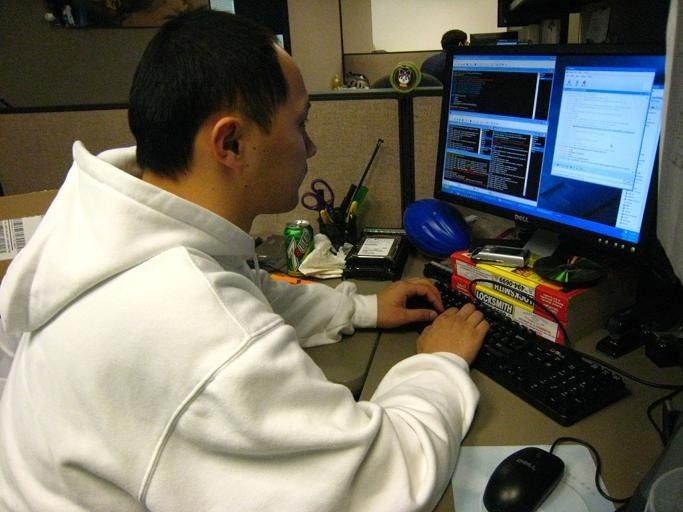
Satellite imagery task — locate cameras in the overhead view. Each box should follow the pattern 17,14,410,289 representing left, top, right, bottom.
477,244,530,268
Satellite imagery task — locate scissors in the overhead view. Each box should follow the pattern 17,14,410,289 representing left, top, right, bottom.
300,179,334,213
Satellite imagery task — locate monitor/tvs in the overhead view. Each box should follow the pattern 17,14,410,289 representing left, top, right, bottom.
470,32,518,46
433,41,666,259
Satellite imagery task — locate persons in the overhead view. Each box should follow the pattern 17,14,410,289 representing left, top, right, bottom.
0,5,491,512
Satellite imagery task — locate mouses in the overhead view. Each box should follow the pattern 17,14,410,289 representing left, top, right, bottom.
483,448,564,512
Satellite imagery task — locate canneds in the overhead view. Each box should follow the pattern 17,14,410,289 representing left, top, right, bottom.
284,219,316,278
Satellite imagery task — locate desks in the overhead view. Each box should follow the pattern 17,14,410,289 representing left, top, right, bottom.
358,252,683,512
256,233,396,396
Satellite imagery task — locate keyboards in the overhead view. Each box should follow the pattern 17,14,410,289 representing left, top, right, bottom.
405,282,626,426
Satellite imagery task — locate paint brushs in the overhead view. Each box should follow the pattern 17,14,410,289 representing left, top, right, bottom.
352,138,384,200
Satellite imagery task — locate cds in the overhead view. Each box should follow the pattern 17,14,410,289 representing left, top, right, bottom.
533,255,604,284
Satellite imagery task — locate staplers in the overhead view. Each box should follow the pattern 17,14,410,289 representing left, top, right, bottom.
595,296,681,359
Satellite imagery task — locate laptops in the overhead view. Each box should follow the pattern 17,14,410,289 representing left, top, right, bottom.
496,40,518,46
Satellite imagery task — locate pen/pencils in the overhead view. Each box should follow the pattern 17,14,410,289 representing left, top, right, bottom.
320,183,369,224
